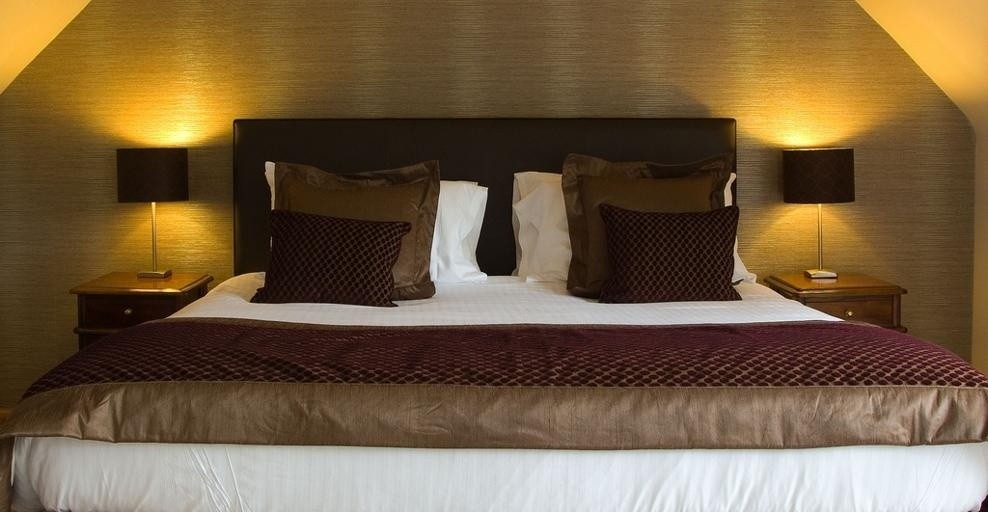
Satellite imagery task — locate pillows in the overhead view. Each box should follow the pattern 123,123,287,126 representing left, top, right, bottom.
269,158,442,299
505,165,758,287
260,154,488,283
594,197,745,308
247,208,415,310
559,148,736,300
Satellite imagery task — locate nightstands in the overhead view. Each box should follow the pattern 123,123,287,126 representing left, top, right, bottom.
764,273,911,336
68,270,213,353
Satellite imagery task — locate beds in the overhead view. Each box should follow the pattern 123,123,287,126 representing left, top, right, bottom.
0,117,987,512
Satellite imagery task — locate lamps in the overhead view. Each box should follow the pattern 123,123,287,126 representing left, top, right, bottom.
111,145,192,277
775,143,858,285
